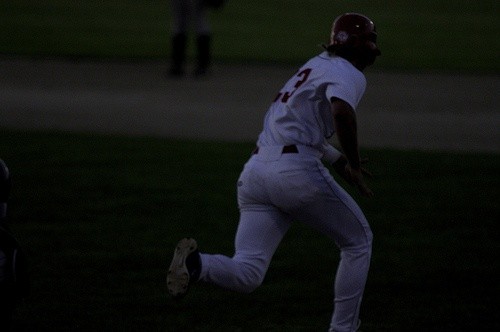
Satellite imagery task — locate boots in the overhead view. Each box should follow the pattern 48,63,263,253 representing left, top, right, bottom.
171,33,185,77
193,35,211,77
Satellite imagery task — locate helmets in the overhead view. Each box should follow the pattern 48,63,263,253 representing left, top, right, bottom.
330,12,377,64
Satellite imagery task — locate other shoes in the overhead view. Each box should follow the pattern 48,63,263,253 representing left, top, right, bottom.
166,237,198,296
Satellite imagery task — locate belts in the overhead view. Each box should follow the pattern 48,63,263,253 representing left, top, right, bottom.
251,144,299,153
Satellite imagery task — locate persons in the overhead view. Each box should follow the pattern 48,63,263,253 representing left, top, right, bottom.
166,14,379,332
169,0,217,78
